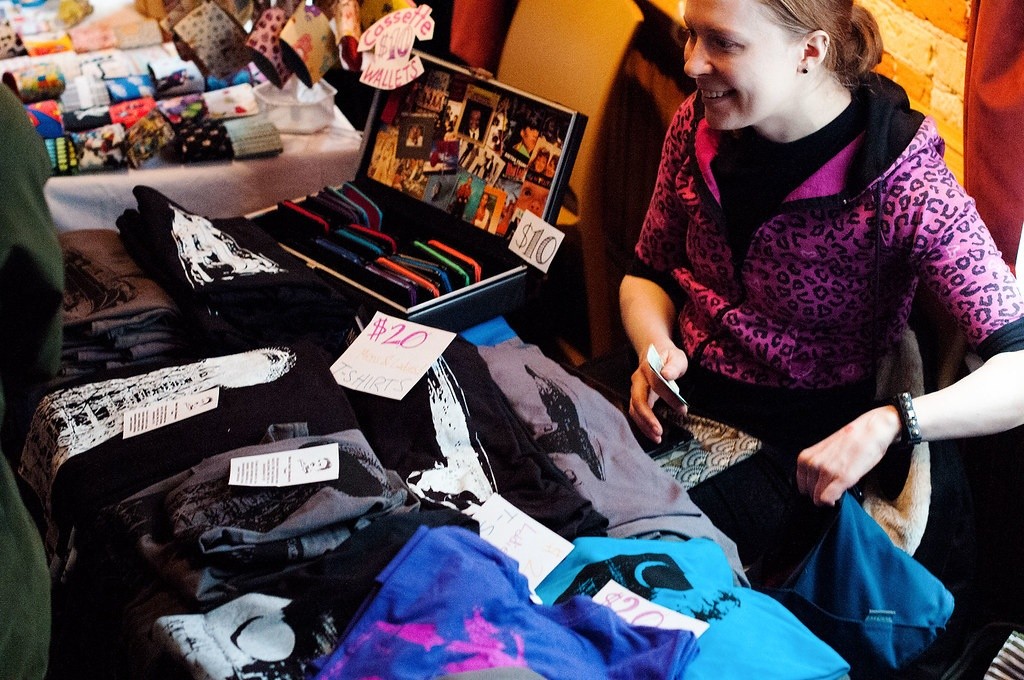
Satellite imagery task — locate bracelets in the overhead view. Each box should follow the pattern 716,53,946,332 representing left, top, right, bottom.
892,393,922,447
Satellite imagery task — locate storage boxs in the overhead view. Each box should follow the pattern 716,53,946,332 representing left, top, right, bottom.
230,44,600,338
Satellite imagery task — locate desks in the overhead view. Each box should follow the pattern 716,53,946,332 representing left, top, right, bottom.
41,94,366,237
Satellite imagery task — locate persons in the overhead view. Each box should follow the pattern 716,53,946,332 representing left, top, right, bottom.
297,455,332,475
467,109,485,143
618,0,1024,557
399,158,425,201
458,139,504,185
512,120,541,157
529,146,560,188
450,176,474,217
406,123,424,148
514,180,550,219
486,116,504,152
0,82,65,680
468,193,491,230
437,105,459,132
542,116,561,144
419,81,450,111
